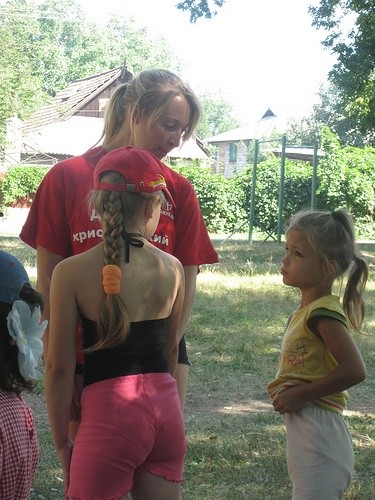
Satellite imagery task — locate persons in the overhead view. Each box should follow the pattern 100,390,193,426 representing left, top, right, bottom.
18,68,219,422
43,144,189,500
267,203,370,500
0,248,49,499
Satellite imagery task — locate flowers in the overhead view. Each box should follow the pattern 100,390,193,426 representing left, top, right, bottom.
6,299,49,381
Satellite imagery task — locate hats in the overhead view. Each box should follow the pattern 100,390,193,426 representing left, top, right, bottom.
0,250,31,305
92,144,176,212
